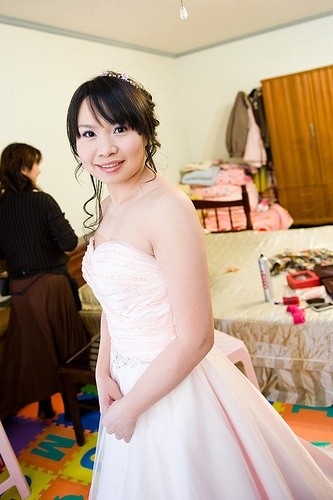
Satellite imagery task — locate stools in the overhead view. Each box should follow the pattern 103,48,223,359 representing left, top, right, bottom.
60,331,103,446
214,328,258,390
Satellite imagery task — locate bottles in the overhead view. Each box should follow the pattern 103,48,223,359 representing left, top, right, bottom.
259,254,272,302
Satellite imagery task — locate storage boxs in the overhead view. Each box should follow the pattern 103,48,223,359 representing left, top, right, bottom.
287,270,320,290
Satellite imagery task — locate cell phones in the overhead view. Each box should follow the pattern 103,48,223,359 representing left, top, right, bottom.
311,302,333,313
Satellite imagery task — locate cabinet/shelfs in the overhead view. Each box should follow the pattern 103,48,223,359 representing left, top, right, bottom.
259,65,333,225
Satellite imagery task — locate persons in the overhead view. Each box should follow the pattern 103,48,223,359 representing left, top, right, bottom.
0,142,89,419
67,73,333,500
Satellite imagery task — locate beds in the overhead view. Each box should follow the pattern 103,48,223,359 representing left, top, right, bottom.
189,164,296,231
0,224,333,407
